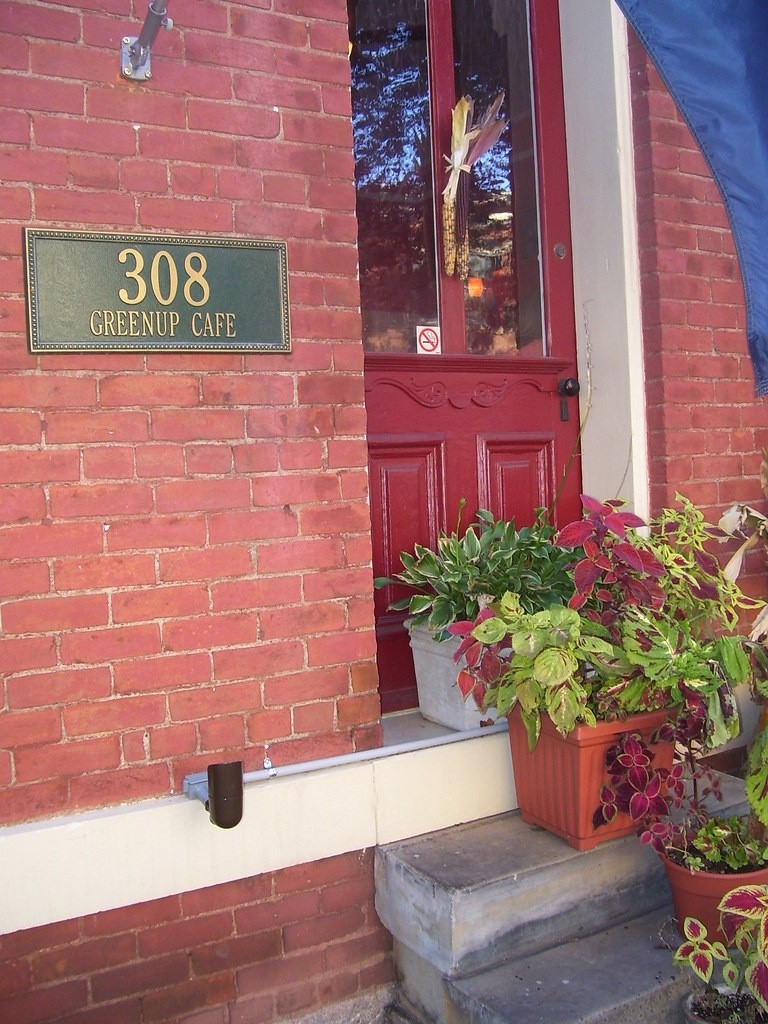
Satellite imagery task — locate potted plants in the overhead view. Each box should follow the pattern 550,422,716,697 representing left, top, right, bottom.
375,497,768,1024
